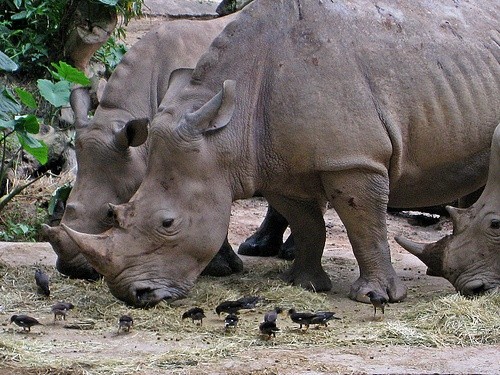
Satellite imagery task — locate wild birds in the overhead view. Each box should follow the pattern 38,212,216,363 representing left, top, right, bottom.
215,296,258,318
117,315,133,335
362,290,390,316
49,302,75,325
182,307,206,327
286,308,341,330
10,315,43,331
225,315,239,332
34,268,50,297
259,307,283,338
